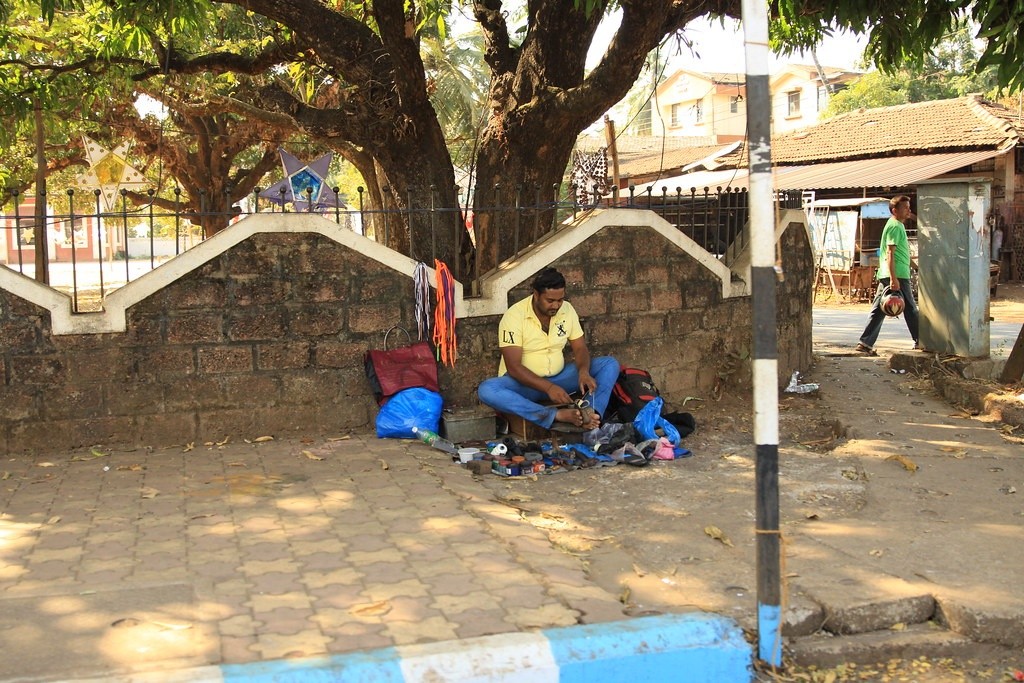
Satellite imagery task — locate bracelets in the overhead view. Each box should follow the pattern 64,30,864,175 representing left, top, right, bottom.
546,383,554,393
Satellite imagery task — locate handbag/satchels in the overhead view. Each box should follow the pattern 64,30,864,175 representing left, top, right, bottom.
376,387,443,439
363,324,440,406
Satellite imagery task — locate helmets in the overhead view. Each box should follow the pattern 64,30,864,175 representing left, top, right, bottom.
880,285,905,316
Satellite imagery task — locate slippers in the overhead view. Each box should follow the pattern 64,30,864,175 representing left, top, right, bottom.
635,438,661,460
607,441,648,467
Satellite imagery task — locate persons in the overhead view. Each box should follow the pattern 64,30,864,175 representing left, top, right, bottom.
857,194,924,354
478,268,620,429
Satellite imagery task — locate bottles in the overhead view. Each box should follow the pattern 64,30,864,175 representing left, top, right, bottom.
797,383,818,393
412,427,454,454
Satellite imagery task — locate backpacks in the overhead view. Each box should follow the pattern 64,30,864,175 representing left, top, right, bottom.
604,366,666,423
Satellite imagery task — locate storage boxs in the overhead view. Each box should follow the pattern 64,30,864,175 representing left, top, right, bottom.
440,406,497,444
502,394,585,442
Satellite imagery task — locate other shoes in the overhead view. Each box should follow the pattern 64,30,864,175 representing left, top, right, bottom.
915,342,919,348
855,342,877,356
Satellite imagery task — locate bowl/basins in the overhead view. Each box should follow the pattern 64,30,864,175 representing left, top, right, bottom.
458,448,480,463
